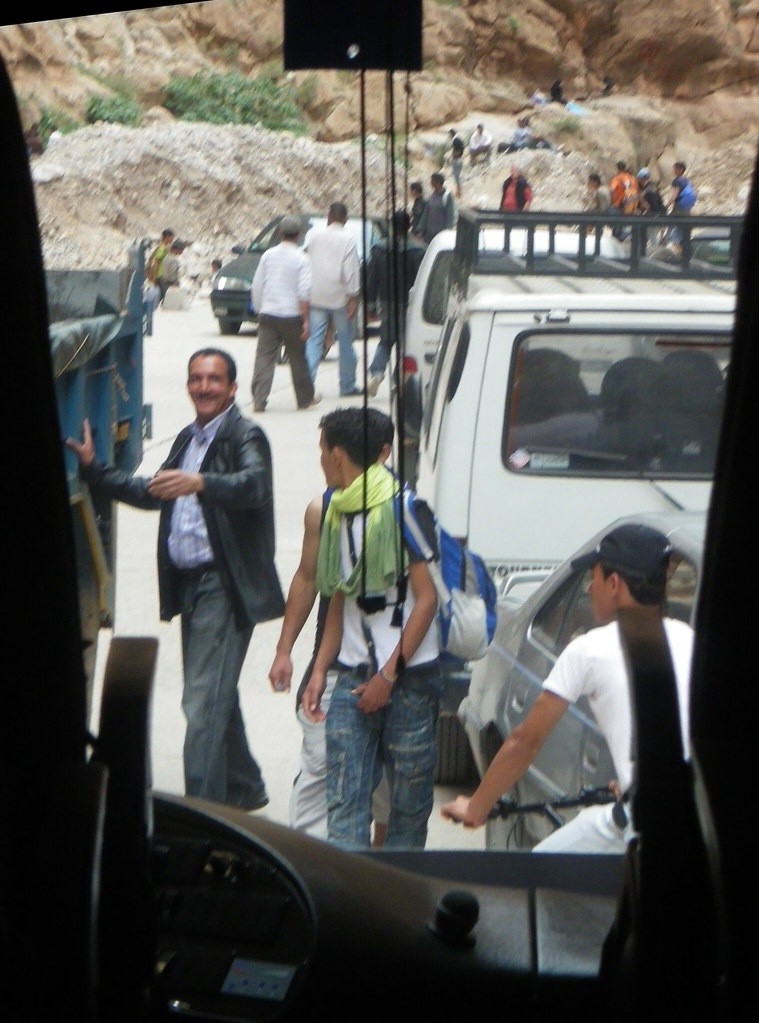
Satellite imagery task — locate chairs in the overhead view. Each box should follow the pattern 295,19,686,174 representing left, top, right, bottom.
517,348,724,425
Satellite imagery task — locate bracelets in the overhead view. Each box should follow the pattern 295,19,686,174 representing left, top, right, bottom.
381,668,397,685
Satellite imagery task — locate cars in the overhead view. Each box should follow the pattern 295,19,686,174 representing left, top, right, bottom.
208,211,400,335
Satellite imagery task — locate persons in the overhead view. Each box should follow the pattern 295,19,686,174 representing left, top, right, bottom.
303,409,445,851
146,229,221,309
364,211,428,398
269,409,393,846
580,160,697,248
302,202,367,396
250,216,323,412
501,168,534,212
441,522,695,853
447,128,466,198
497,78,569,154
67,348,287,813
466,124,495,166
410,174,456,243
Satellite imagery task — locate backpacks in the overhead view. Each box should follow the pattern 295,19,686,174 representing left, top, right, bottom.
322,484,496,669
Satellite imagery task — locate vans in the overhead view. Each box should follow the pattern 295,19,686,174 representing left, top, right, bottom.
393,227,625,488
399,268,738,782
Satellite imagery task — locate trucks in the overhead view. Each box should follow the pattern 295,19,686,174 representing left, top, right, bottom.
46,234,152,716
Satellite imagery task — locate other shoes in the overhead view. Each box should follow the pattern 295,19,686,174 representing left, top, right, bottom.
306,393,321,408
253,402,266,412
346,387,363,395
233,786,270,812
366,373,384,396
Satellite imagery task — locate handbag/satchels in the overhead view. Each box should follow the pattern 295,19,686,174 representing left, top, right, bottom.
675,178,696,212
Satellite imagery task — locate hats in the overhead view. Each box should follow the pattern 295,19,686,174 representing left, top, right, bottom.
636,168,649,179
571,524,671,573
281,214,304,236
